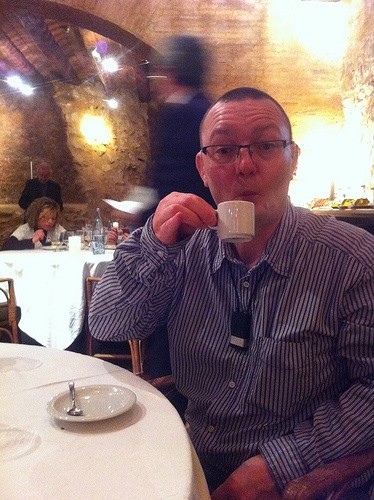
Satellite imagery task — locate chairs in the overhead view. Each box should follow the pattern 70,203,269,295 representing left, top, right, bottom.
145,375,374,500
86,275,145,376
0,277,20,344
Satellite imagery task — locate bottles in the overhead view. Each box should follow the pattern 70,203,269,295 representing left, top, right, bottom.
91,208,105,235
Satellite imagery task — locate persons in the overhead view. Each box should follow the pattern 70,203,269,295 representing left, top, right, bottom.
18,161,64,224
87,87,374,500
0,197,73,251
139,34,215,225
102,186,160,246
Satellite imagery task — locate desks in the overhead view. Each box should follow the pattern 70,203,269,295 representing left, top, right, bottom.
0,248,116,350
312,208,374,234
1,341,211,500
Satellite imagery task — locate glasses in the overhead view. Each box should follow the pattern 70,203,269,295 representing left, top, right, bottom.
199,139,292,165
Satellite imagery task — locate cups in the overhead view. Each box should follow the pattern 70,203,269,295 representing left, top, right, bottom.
208,201,255,243
62,230,92,246
68,236,82,254
92,235,105,254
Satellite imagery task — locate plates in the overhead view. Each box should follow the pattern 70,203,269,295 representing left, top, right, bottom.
105,244,116,250
47,383,136,422
40,246,67,250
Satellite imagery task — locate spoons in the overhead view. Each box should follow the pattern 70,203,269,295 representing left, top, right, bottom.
66,380,83,416
